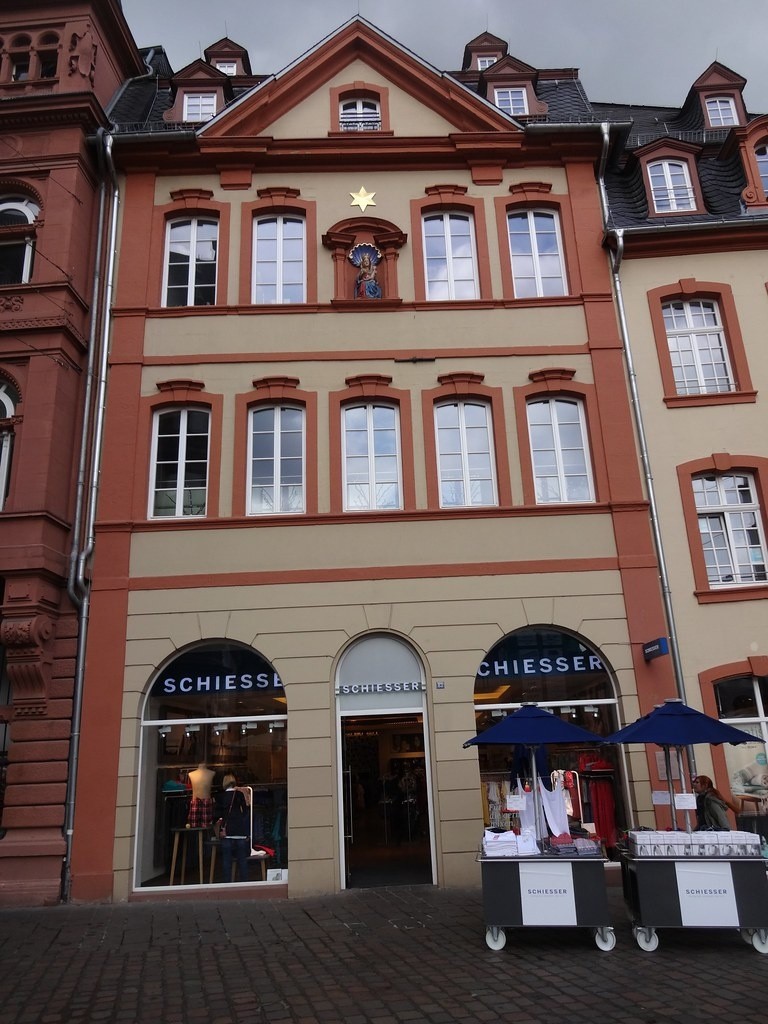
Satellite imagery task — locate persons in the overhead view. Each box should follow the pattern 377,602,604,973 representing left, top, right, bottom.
693,775,740,830
360,252,376,273
213,774,250,882
188,763,216,828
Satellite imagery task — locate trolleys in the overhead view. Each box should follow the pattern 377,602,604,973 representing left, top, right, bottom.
615,841,767,954
475,847,616,953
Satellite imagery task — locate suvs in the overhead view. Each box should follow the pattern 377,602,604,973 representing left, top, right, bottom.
154,761,287,830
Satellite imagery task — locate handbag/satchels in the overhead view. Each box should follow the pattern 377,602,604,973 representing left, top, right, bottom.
212,818,227,838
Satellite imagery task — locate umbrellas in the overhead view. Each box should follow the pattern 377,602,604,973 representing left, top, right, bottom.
464,702,604,842
606,698,766,832
